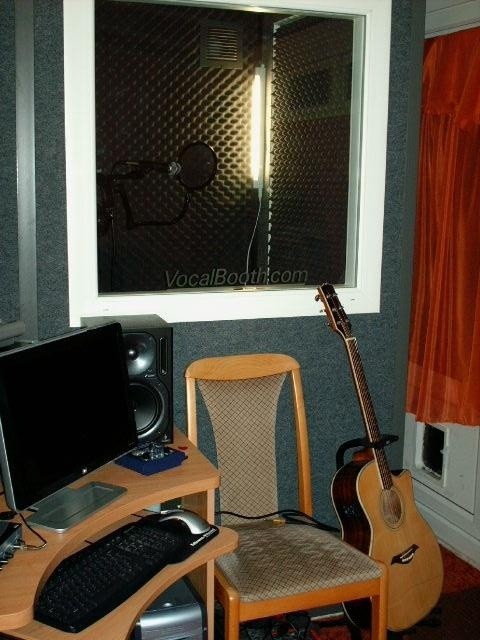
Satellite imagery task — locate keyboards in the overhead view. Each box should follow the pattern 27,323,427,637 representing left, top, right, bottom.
36,522,187,634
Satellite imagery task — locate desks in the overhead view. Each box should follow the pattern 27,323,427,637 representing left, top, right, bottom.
0,424,239,640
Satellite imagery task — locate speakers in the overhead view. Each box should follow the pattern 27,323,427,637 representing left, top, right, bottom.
79,314,174,452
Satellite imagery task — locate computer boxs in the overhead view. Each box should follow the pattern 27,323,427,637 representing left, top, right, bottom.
131,578,204,640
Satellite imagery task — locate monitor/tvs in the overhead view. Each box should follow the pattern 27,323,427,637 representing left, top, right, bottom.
0,320,140,535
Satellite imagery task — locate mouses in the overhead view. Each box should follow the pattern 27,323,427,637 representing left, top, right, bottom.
156,509,212,537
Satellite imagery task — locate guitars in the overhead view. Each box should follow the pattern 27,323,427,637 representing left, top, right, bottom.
315,283,444,636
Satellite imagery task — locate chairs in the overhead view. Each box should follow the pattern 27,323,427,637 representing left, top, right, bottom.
185,353,389,639
